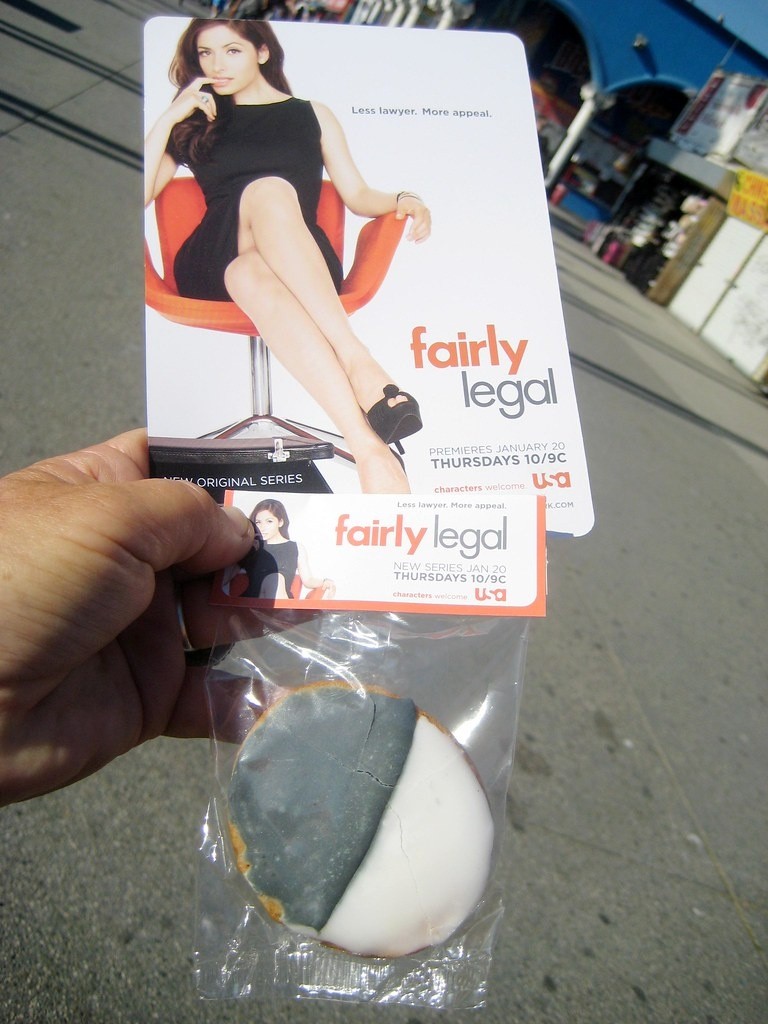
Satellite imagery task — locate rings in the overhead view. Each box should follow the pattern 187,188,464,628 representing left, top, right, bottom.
202,96,209,102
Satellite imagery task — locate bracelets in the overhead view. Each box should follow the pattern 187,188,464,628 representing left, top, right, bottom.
397,191,420,201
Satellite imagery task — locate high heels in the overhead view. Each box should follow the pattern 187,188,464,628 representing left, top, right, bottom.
365,384,422,454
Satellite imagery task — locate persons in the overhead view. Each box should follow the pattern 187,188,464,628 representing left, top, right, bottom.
0,427,336,807
143,18,432,494
222,499,329,599
